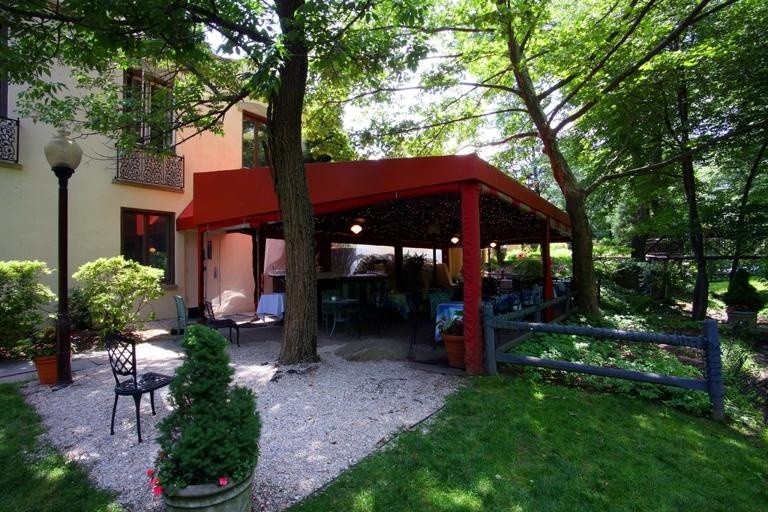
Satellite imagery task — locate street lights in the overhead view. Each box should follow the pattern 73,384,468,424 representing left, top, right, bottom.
43,126,83,384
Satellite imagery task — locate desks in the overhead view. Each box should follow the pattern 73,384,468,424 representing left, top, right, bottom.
256,293,286,326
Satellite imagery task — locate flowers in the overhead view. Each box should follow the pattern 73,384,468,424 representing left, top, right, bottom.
146,322,263,498
433,310,464,351
30,326,58,361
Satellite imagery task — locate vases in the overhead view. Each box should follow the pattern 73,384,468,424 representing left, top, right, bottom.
161,470,254,512
441,332,465,369
34,355,58,384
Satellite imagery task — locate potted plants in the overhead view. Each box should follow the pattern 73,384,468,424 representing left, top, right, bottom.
709,268,767,332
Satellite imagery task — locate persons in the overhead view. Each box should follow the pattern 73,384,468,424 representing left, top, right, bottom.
448,269,464,302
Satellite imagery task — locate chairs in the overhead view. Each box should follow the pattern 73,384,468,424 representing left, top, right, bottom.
105,329,176,443
205,300,240,347
172,295,204,346
